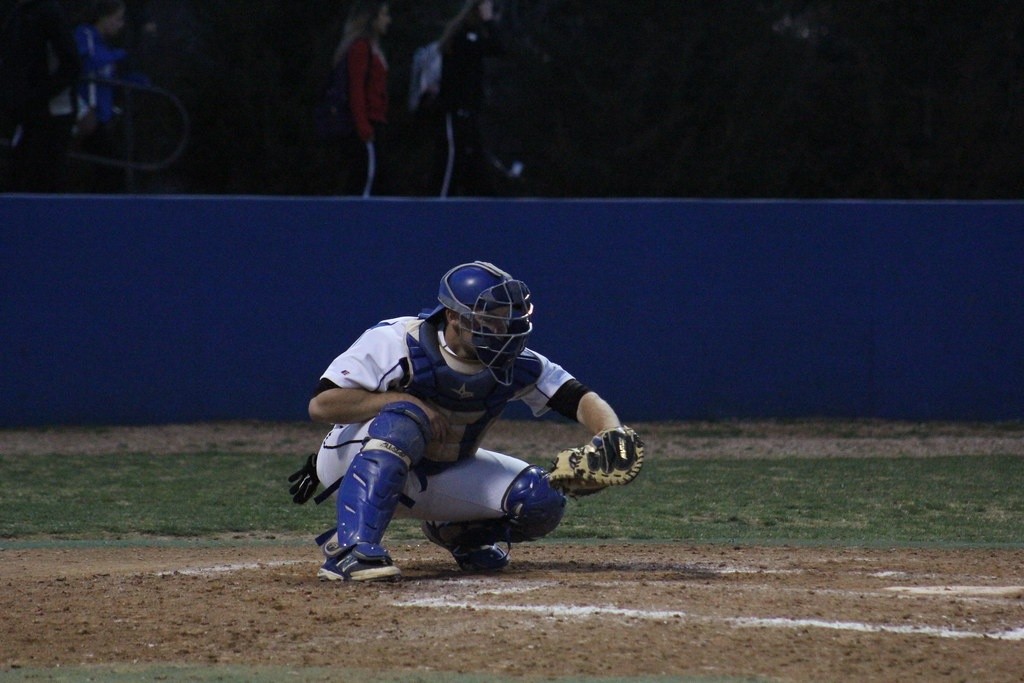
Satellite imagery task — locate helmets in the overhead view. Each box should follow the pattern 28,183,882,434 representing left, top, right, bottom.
425,261,516,321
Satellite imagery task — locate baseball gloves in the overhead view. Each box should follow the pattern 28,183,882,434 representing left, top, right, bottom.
547,424,645,497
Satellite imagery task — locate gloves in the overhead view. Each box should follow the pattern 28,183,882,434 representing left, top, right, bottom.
288,453,320,505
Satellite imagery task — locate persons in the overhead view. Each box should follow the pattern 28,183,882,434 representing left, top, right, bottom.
0,0,152,195
337,0,393,200
308,259,644,583
407,0,527,198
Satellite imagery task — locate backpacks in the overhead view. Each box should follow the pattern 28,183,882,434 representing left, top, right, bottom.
312,53,352,141
408,37,444,121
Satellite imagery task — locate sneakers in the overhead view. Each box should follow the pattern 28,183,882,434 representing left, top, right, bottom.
421,519,503,575
317,542,401,584
